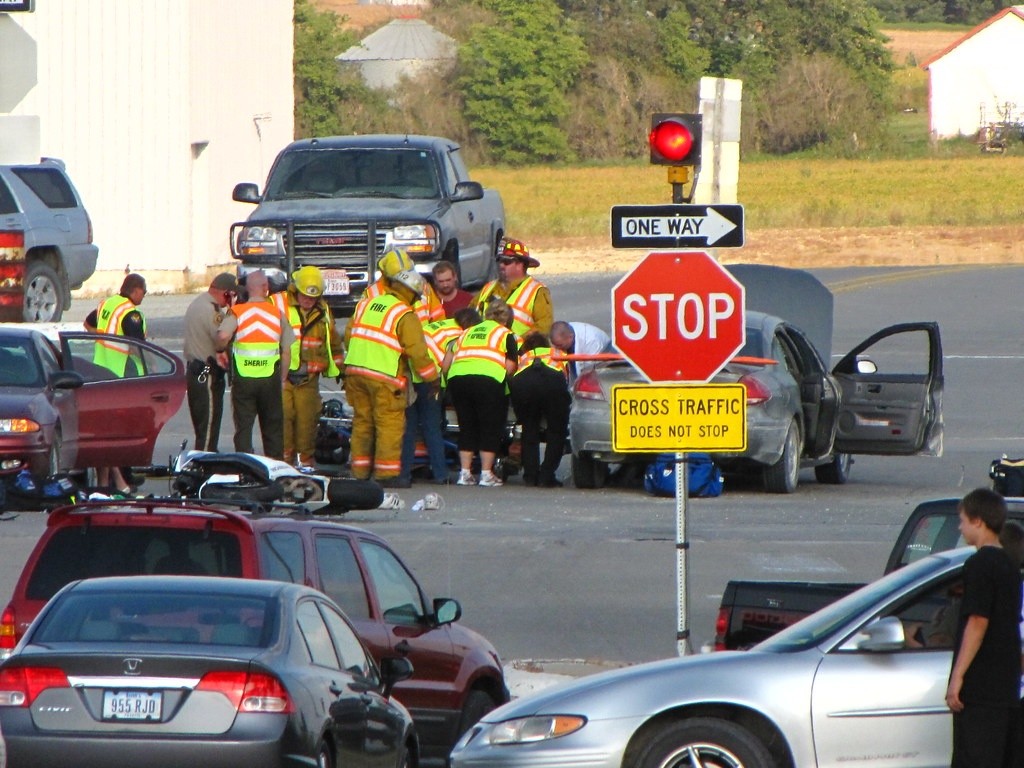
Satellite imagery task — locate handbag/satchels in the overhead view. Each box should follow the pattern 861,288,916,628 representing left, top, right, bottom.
643,450,725,498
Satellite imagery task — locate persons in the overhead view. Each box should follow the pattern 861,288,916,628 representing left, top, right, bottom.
181,273,235,452
268,264,344,473
944,487,1024,768
344,269,439,488
18,343,132,497
344,248,446,463
84,274,150,491
399,237,611,488
212,269,293,462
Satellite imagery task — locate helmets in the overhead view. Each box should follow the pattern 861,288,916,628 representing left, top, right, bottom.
292,265,325,297
378,247,415,282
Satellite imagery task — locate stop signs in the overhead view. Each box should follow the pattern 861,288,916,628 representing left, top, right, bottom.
611,249,746,384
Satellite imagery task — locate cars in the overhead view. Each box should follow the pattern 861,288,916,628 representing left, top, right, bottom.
0,573,421,768
569,308,945,493
0,495,512,763
447,544,1024,768
1,329,187,508
1,156,99,325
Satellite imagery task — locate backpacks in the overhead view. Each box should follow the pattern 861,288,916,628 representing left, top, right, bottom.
0,469,136,517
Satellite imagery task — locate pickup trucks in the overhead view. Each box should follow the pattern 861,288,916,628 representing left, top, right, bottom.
231,133,507,315
711,492,1024,651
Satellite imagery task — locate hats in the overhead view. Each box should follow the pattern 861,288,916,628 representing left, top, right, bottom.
210,272,242,297
392,269,424,300
494,238,540,269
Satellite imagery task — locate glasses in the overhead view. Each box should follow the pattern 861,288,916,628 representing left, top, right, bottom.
141,287,147,295
497,257,521,265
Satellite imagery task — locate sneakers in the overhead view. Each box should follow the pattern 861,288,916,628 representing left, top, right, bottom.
479,472,504,486
456,471,477,485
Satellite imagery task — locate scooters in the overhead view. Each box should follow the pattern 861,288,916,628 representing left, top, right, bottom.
165,438,385,519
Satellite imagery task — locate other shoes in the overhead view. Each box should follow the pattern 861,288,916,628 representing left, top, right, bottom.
436,470,458,485
525,480,535,487
374,475,411,488
123,486,146,498
536,480,563,488
